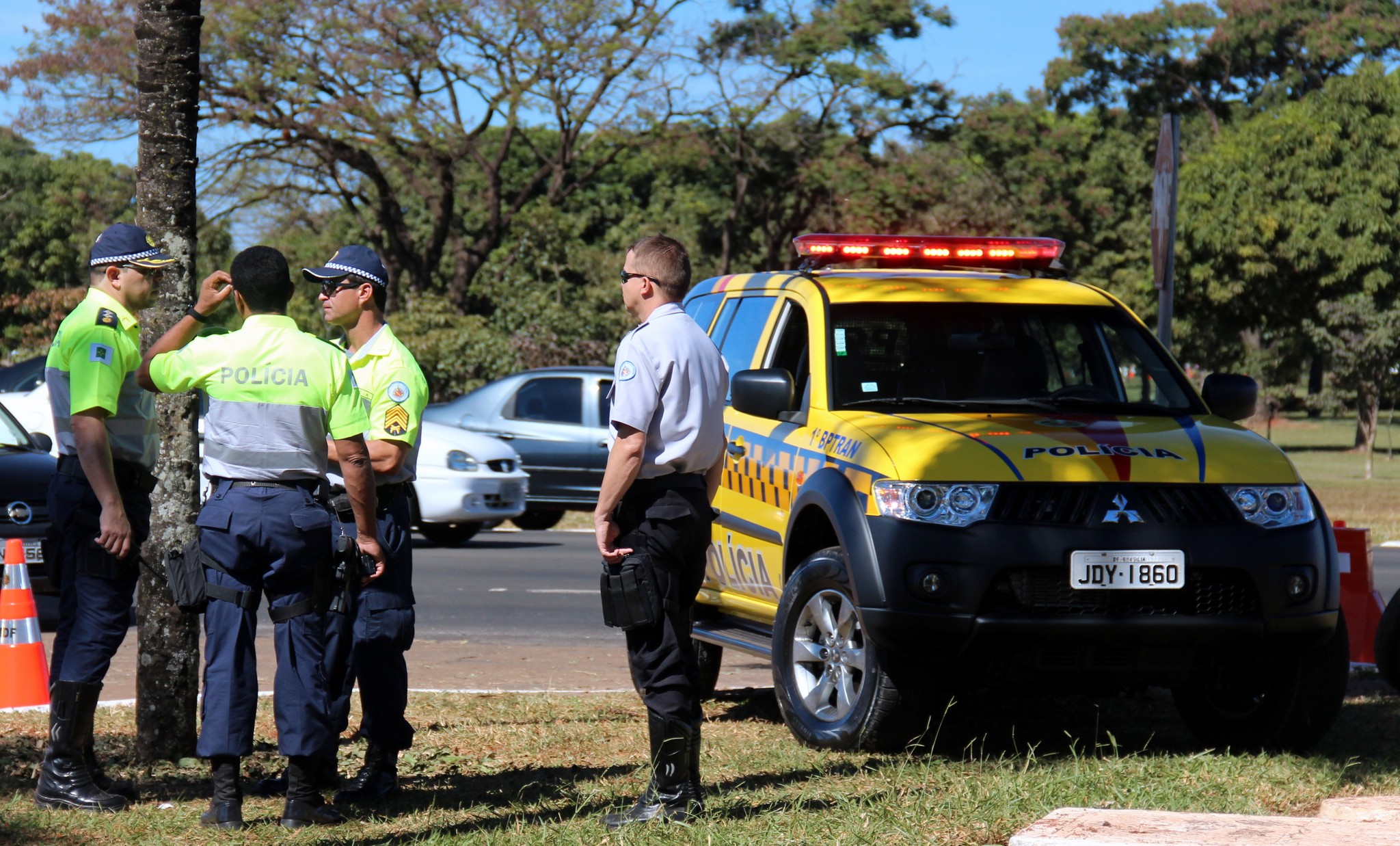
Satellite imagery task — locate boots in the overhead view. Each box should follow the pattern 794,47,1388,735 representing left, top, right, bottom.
280,754,349,828
199,755,244,831
597,708,704,828
33,676,132,813
333,748,400,806
259,752,338,793
688,723,704,817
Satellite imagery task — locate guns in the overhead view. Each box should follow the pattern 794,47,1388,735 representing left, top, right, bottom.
327,534,378,613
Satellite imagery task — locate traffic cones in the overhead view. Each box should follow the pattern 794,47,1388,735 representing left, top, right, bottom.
0,535,50,714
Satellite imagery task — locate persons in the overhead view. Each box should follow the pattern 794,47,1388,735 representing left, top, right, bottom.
133,245,385,831
35,222,181,813
594,237,728,828
301,246,430,817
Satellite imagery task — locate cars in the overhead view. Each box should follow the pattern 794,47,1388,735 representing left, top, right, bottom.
198,397,530,549
0,352,65,634
678,230,1352,757
420,364,614,530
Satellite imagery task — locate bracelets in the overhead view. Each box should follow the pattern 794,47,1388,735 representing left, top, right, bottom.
183,304,207,324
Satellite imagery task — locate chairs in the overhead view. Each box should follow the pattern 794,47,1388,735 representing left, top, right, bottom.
956,330,1050,398
798,327,871,411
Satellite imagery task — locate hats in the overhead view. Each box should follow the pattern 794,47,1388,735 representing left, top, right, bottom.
301,244,389,289
88,221,180,268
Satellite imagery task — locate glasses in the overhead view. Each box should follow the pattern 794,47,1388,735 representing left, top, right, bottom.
621,269,662,286
322,279,365,297
